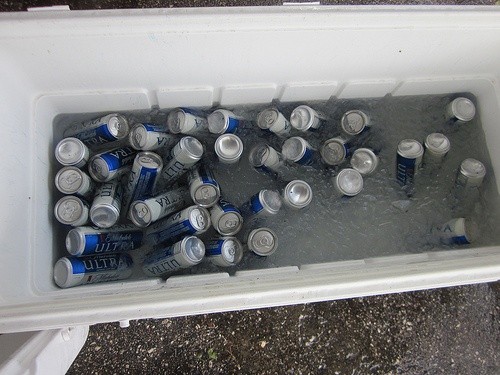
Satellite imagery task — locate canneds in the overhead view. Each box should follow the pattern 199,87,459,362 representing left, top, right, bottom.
395,138,425,199
423,132,450,172
432,218,475,247
53,106,381,290
440,97,476,127
454,158,486,204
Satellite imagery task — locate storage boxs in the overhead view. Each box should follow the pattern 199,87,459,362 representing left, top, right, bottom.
0,2,500,334
